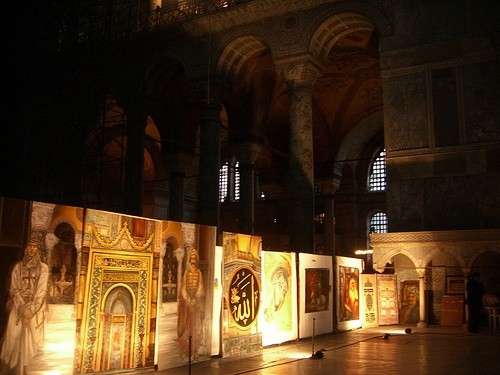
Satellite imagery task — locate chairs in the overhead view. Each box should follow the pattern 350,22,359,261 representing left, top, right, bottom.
485,307,500,331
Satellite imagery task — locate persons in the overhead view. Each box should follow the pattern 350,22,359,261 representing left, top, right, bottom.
467,272,486,334
